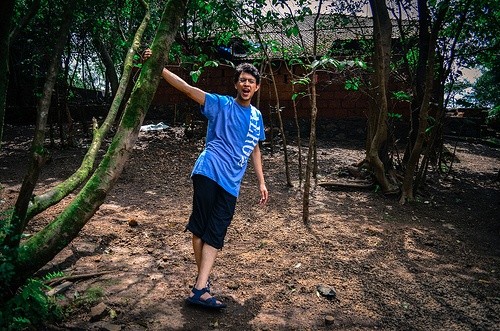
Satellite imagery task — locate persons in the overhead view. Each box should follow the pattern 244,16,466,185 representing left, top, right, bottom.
139,48,268,310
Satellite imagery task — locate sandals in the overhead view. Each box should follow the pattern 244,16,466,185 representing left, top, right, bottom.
186,286,226,308
192,276,211,293
